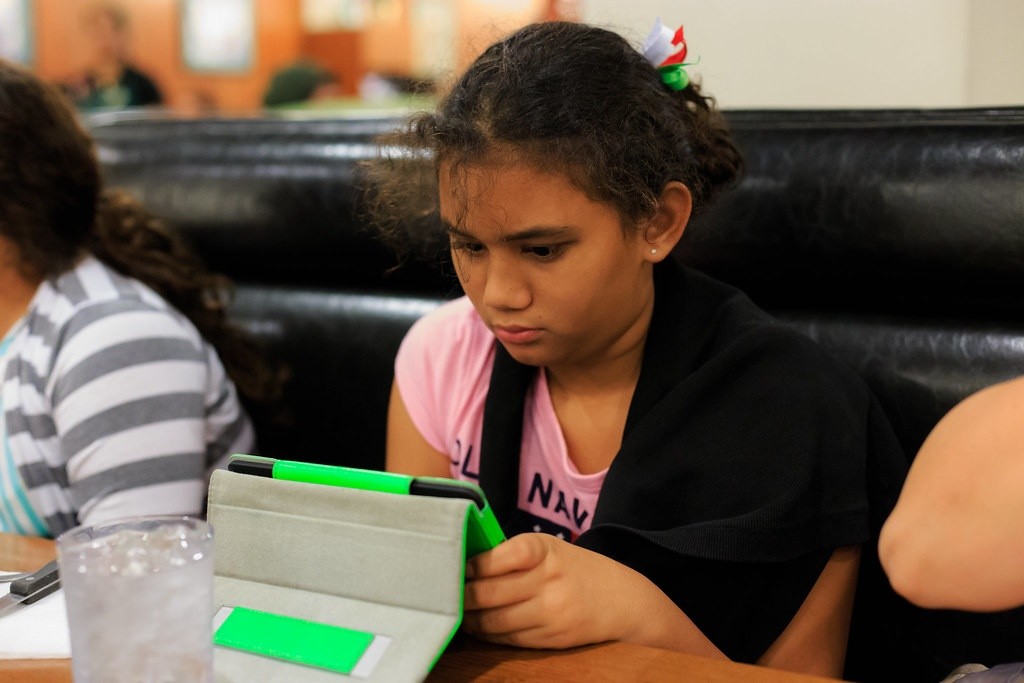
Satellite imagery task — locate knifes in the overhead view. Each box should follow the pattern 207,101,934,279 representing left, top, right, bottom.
1,558,59,616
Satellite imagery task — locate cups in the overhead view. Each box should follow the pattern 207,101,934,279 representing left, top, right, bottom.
58,515,216,683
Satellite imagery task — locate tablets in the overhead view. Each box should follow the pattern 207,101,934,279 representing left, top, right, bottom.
227,454,510,557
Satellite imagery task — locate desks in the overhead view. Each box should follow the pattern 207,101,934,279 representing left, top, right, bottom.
1,531,846,683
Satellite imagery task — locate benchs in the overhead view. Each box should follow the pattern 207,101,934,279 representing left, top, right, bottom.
84,103,1024,683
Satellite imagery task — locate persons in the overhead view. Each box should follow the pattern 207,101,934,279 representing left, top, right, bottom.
379,18,894,683
1,58,253,553
878,373,1021,612
